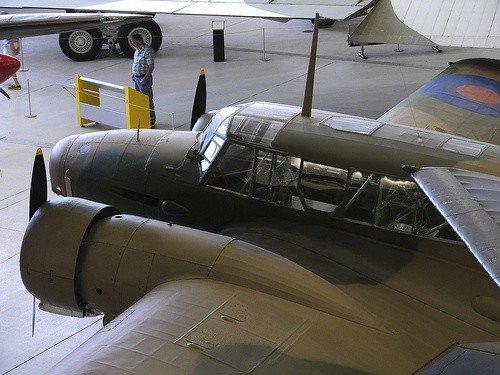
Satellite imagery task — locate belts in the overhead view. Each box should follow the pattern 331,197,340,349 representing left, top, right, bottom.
133,73,147,78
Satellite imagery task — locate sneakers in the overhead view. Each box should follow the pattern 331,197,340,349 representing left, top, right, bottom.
8,84,22,90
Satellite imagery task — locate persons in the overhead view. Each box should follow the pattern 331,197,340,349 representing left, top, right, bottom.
130,34,156,129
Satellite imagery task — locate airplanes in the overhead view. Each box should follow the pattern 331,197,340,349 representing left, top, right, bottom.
23,52,499,374
